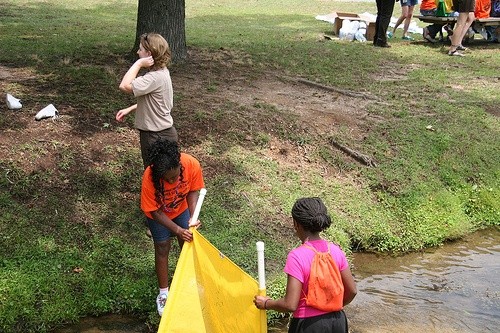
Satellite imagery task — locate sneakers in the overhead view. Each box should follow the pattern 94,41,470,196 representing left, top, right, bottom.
35,104,58,120
156,294,167,316
426,35,436,42
423,28,429,39
439,35,443,41
6,94,22,109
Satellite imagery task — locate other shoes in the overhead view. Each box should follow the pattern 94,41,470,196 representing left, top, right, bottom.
374,43,391,47
456,45,467,50
403,36,415,41
389,32,392,37
448,50,463,55
147,229,177,237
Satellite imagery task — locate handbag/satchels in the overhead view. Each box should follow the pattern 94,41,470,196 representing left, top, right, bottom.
435,1,445,16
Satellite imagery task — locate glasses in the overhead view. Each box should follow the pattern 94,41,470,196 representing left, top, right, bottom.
144,36,149,45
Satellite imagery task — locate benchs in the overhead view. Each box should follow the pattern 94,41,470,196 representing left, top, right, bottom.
413,15,500,43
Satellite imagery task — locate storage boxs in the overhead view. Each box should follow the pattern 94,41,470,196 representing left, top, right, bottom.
365,22,377,41
334,12,365,36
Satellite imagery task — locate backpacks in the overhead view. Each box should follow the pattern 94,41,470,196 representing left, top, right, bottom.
302,243,344,312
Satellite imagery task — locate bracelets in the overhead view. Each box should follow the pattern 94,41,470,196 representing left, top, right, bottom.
265,299,272,310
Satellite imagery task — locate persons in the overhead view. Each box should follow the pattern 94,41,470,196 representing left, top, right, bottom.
140,138,206,317
375,0,500,57
253,197,357,333
116,32,178,237
